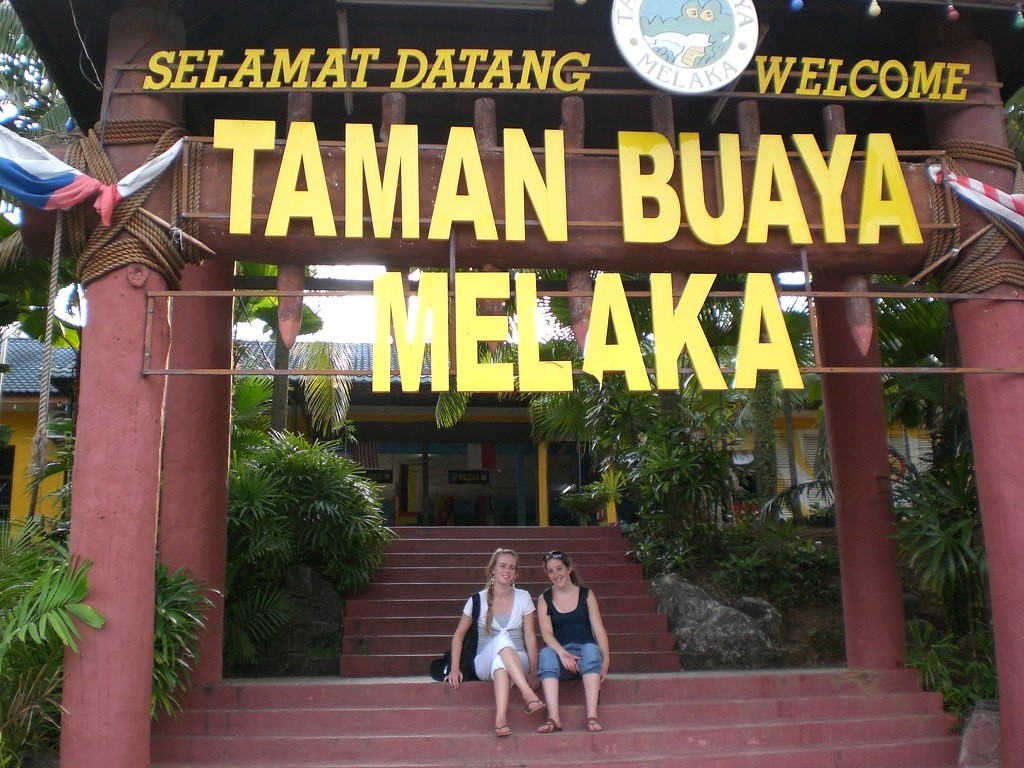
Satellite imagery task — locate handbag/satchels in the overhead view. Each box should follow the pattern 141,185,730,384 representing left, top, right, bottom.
430,592,481,682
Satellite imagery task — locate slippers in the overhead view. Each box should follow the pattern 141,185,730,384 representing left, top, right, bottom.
494,725,512,736
524,699,545,714
586,717,602,732
538,719,563,733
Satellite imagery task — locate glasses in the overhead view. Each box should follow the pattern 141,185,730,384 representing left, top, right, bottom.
542,552,564,568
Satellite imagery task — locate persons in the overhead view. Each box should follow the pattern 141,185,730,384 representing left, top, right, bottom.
536,550,610,734
443,547,546,737
